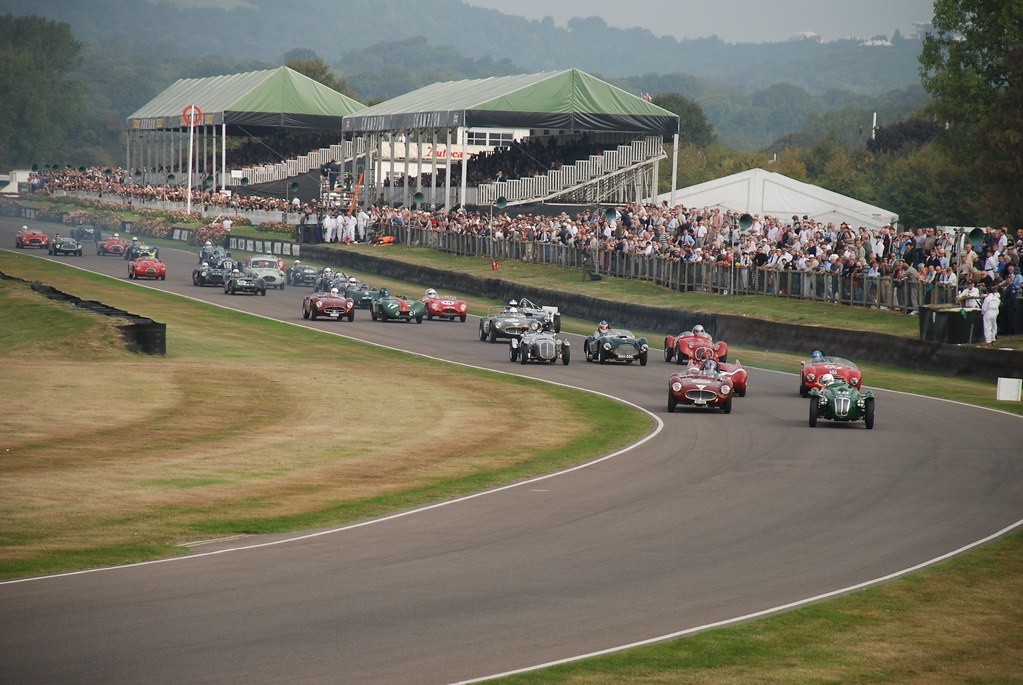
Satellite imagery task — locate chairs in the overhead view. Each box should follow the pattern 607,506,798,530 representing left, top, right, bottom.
688,367,701,374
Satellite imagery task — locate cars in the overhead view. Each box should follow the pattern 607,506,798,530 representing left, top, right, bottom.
668,358,735,413
72,223,103,242
808,373,878,431
479,302,543,343
128,251,168,280
49,235,83,257
584,326,650,366
287,259,424,326
127,242,160,261
242,254,288,290
191,240,242,288
507,320,574,366
301,289,356,323
97,236,128,257
17,229,50,250
421,292,468,323
517,298,563,333
689,354,748,395
220,263,267,296
663,325,729,363
799,348,864,395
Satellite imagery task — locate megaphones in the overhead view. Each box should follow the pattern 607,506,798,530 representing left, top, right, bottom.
734,214,753,229
494,196,507,208
288,181,299,192
31,163,175,180
412,192,424,200
599,207,617,220
204,176,213,184
962,227,984,246
238,177,248,185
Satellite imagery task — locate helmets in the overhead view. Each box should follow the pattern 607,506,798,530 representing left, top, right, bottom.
599,320,609,333
426,289,436,298
507,299,518,308
379,288,388,297
331,287,338,295
812,350,822,360
705,361,715,372
349,277,357,285
692,325,705,335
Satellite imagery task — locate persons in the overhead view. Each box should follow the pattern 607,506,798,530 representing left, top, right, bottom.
510,308,518,318
383,136,617,189
398,133,405,142
509,299,517,306
22,225,28,232
152,132,353,173
812,350,821,361
822,374,834,387
982,286,999,347
594,321,608,339
693,325,704,334
428,289,436,299
705,361,715,372
29,167,1023,317
700,352,710,361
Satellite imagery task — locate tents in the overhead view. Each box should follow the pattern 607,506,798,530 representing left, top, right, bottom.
125,65,369,191
341,68,680,211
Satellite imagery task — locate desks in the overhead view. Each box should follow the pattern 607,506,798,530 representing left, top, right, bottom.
918,304,985,345
